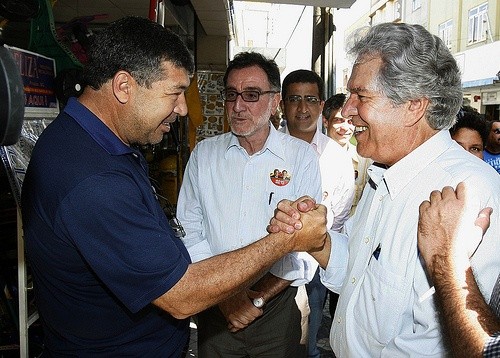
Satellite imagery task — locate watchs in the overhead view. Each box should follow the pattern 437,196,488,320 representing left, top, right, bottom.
249,297,267,310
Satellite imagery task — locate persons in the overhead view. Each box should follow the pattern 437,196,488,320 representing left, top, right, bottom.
22,16,327,358
183,23,500,358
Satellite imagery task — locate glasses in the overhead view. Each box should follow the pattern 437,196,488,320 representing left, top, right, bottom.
220,87,279,102
156,194,186,238
284,94,320,105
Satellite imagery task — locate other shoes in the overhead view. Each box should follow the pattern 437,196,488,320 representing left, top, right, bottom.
316,337,332,351
